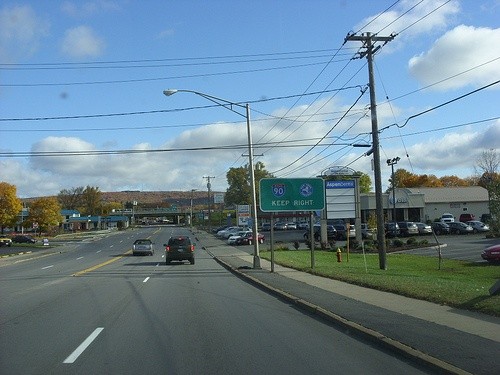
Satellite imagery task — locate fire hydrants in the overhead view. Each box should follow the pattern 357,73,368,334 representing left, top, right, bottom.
335,248,343,262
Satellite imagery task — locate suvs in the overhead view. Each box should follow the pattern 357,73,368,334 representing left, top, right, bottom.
460,214,474,224
440,213,456,223
479,214,493,223
164,236,195,265
385,222,400,238
361,224,378,238
430,222,450,235
334,225,357,237
334,226,346,240
304,225,337,240
397,222,419,236
447,222,473,234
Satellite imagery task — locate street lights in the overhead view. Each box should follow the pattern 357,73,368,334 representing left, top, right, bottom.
164,89,262,270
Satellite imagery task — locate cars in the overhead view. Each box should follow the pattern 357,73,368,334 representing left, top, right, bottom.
263,222,272,231
466,221,490,233
287,222,296,230
221,228,252,238
299,220,309,229
217,227,241,237
132,240,154,255
228,231,251,244
213,226,230,232
273,223,287,231
236,232,265,245
12,235,38,243
417,222,432,235
481,244,500,262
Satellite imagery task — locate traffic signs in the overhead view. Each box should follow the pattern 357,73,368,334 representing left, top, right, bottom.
258,177,326,212
